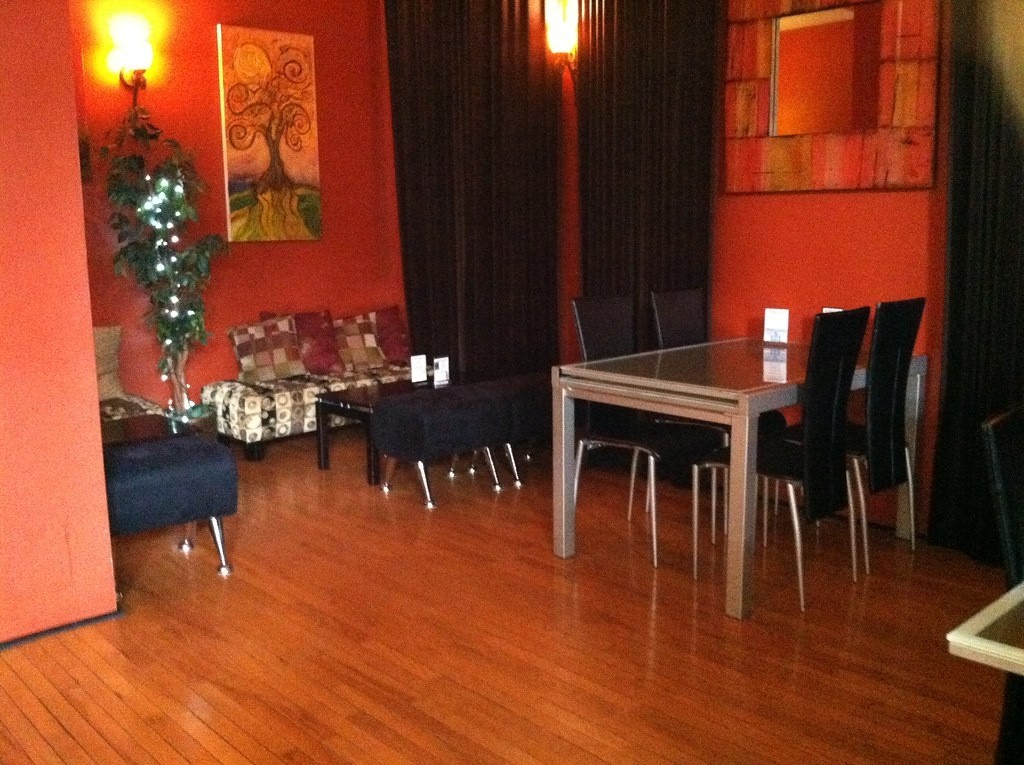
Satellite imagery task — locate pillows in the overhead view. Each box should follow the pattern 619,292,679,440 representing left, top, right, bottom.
332,312,390,371
260,309,346,376
376,305,411,368
227,317,305,378
93,325,123,405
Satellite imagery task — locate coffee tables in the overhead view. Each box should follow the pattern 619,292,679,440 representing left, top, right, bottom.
315,374,494,485
101,413,205,449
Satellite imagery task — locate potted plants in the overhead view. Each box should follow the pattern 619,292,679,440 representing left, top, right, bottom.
101,106,228,444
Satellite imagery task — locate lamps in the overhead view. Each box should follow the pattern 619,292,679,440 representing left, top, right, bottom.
99,16,152,106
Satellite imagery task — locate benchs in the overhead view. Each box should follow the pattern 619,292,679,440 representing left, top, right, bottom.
200,361,435,461
467,373,554,487
104,433,237,577
100,396,164,423
370,380,506,509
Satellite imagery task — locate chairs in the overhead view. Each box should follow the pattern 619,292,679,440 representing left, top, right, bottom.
572,294,717,567
693,305,870,610
776,296,926,580
644,289,787,512
980,403,1024,589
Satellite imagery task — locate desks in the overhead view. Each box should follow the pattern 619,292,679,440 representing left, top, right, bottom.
551,338,932,617
947,582,1024,765
